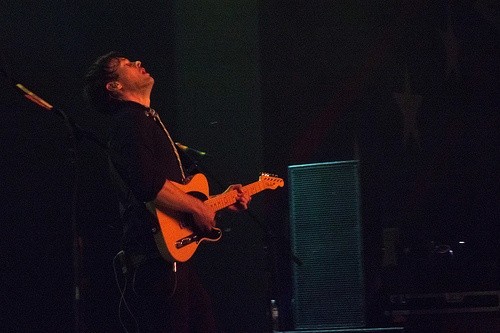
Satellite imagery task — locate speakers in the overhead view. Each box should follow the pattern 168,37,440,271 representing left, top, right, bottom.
286,159,368,330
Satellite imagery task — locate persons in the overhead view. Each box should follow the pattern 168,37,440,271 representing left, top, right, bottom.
85,49,252,333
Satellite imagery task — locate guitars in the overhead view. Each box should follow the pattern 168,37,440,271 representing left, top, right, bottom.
150,172,285,263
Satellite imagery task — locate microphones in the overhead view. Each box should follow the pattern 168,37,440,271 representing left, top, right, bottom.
174,143,208,158
16,85,66,118
